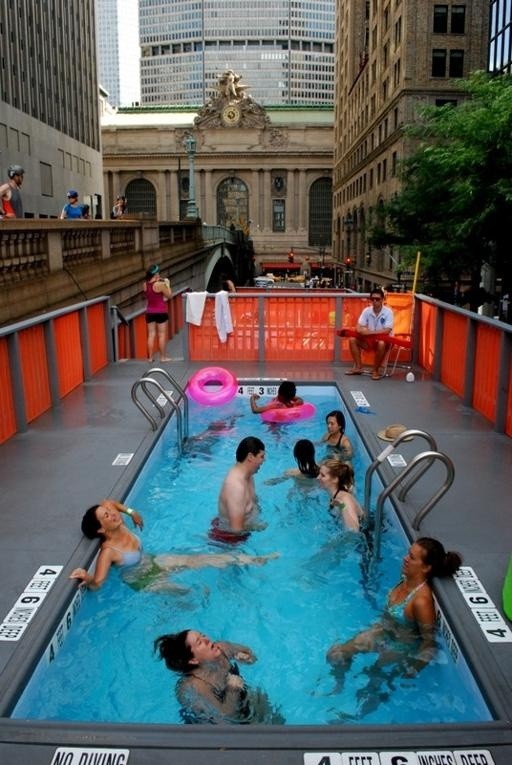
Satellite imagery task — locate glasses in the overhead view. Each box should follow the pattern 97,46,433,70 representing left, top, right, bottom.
370,297,381,302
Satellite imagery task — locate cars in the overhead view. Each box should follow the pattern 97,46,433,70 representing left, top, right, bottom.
255,272,332,289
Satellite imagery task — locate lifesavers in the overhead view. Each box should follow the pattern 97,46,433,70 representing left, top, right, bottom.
261,399,316,423
189,366,236,404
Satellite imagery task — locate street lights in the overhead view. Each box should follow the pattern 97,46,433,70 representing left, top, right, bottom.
235,194,241,227
184,134,201,220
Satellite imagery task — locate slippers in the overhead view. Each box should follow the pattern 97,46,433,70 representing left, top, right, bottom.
345,370,362,375
372,370,381,381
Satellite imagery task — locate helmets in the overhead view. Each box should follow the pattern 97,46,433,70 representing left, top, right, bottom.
8,165,24,179
67,190,79,198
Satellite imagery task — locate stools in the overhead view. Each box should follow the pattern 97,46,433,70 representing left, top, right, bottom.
356,328,394,380
389,330,417,377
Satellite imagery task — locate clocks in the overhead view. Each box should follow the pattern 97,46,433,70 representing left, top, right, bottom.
221,104,243,126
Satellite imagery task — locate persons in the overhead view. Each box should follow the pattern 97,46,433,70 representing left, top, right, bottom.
60,190,83,219
0,164,25,220
80,204,90,218
345,290,394,380
143,263,173,363
218,273,236,293
225,71,238,104
112,195,128,219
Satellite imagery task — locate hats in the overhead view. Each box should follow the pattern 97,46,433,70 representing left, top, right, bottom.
376,422,415,443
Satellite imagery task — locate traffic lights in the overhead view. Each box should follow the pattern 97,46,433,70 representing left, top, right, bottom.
291,252,294,263
347,258,350,265
288,252,290,262
